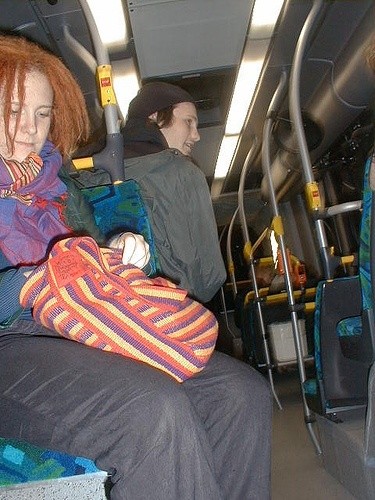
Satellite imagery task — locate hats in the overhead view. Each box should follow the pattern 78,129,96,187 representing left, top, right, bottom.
127,82,194,119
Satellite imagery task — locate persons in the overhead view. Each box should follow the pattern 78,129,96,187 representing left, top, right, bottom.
94,81,226,303
0,36,274,499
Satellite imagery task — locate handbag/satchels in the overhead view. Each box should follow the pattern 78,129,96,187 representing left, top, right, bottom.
20,235,219,383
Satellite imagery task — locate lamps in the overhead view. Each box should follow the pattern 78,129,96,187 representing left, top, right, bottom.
210,0,286,200
85,0,142,126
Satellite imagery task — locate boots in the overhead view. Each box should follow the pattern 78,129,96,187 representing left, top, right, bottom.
242,342,259,368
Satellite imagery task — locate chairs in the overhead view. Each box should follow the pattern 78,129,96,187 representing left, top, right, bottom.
302,274,372,416
0,435,108,499
334,154,375,366
78,179,157,280
